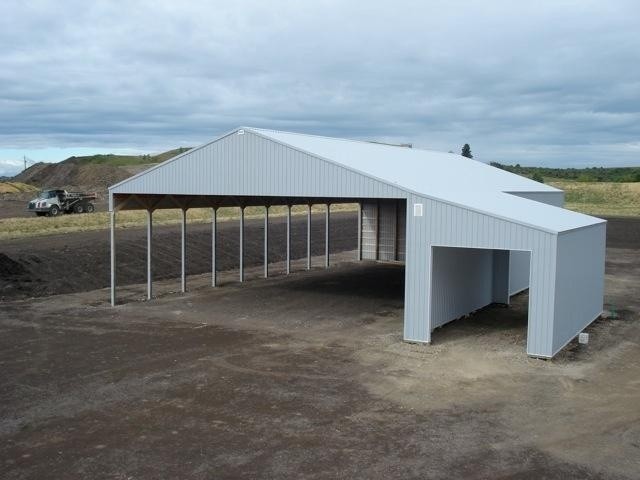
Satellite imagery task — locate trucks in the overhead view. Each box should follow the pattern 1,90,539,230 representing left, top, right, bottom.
25,189,97,217
